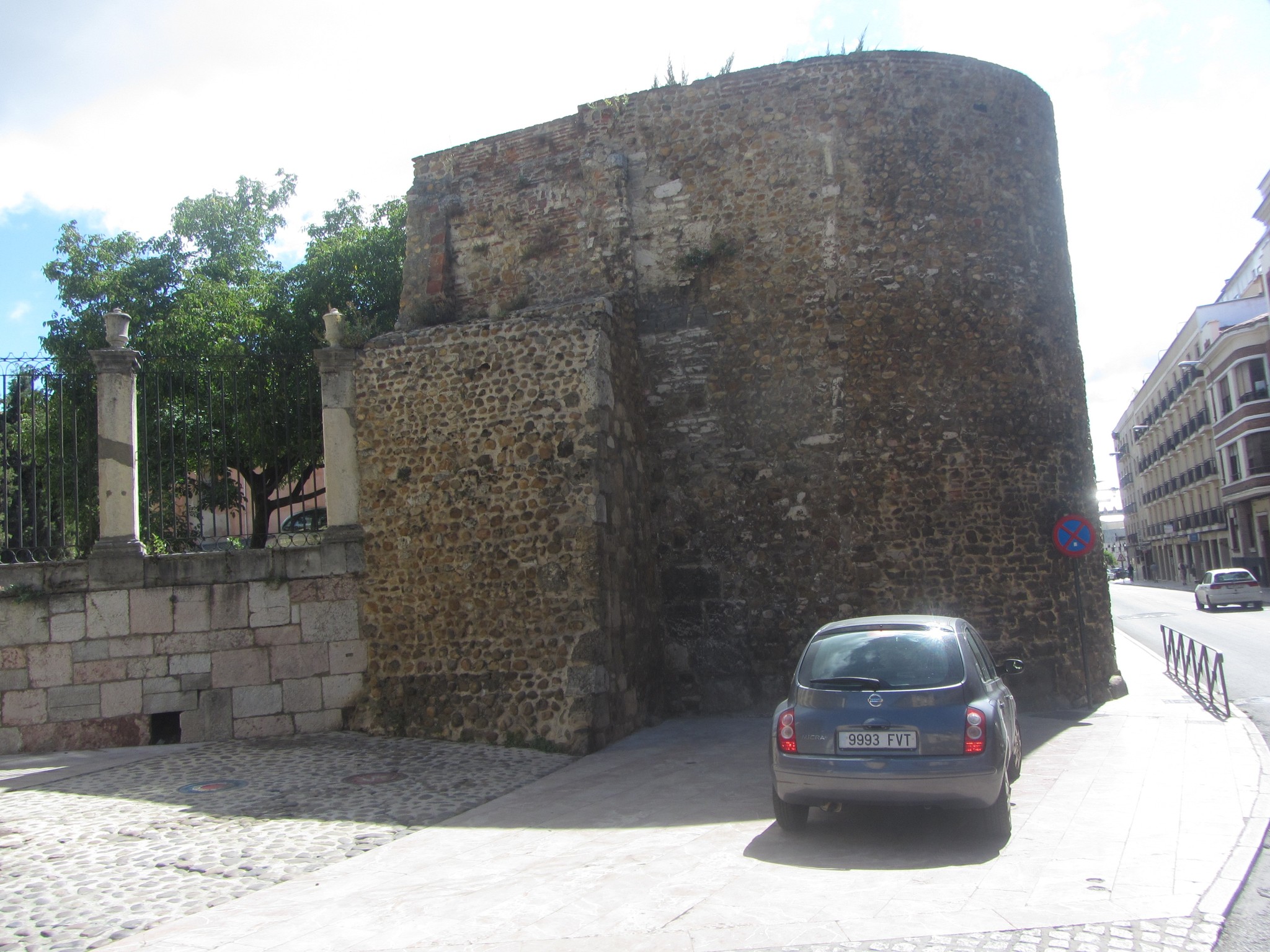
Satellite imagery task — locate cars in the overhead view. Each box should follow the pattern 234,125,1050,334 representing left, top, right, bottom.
1110,568,1129,579
768,615,1025,837
1194,567,1263,612
1107,569,1116,581
